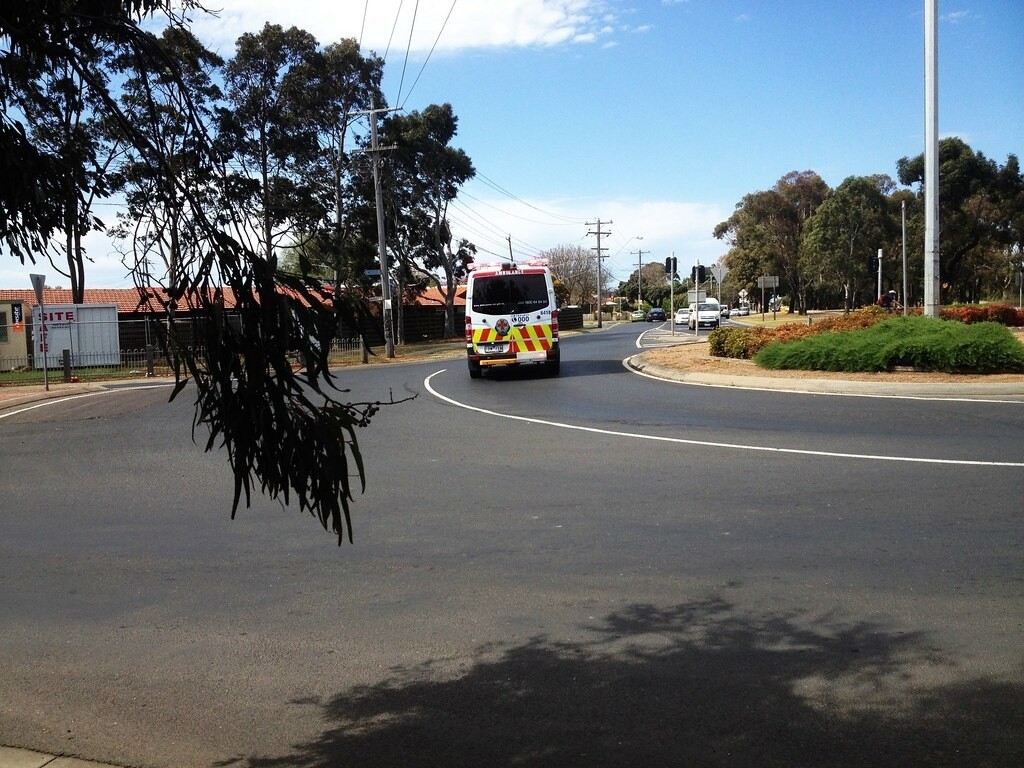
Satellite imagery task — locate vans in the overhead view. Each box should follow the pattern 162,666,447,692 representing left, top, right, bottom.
688,298,719,330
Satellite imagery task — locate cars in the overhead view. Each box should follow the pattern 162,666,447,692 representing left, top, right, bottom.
730,309,739,316
721,305,730,319
631,311,647,322
739,307,749,316
647,308,666,323
674,308,693,325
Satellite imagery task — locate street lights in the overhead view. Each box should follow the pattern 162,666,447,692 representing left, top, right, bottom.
878,249,882,299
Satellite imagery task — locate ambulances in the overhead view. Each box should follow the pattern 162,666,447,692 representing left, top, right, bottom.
465,259,564,379
